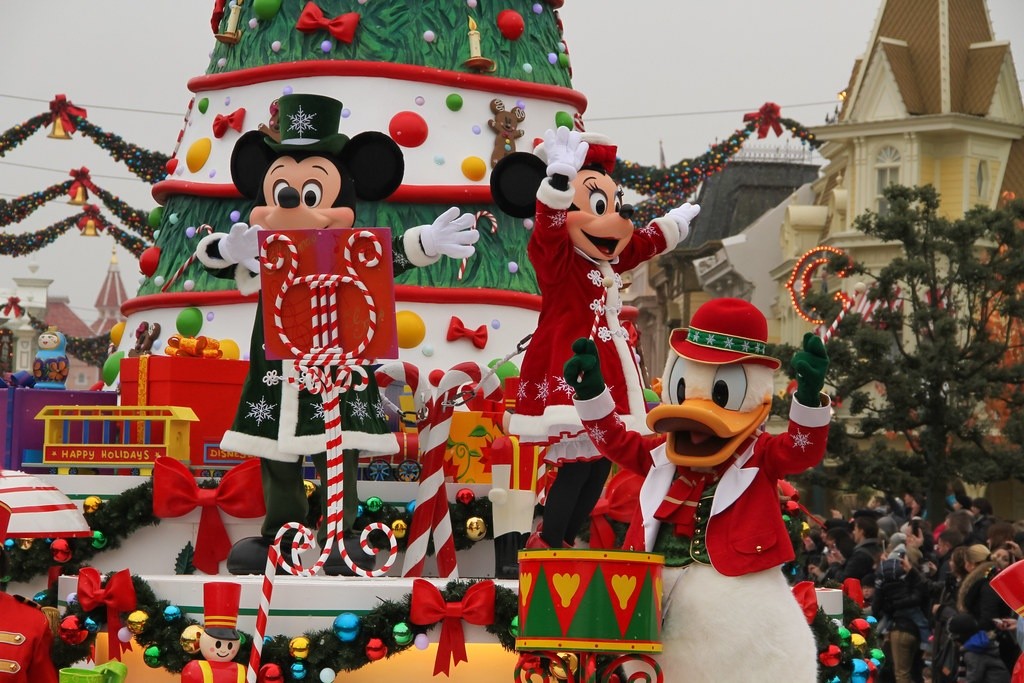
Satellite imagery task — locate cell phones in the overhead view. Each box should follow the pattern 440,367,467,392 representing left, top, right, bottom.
1005,544,1012,550
899,552,905,565
833,543,836,549
993,618,1011,628
913,520,919,538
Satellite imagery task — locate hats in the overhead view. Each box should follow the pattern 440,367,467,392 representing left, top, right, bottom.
0,500,12,550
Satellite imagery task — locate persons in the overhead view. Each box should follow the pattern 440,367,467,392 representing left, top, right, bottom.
180,582,249,683
786,489,1024,683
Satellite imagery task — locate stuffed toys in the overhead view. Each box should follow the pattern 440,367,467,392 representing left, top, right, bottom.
490,126,700,549
562,299,832,683
196,93,480,576
